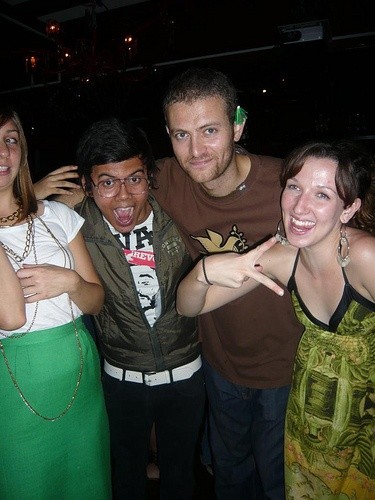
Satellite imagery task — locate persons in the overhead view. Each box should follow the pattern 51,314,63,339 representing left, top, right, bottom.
0,101,112,500
55,66,306,500
31,120,203,500
33,165,82,201
176,143,375,500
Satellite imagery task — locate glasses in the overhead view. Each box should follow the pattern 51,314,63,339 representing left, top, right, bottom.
86,173,151,197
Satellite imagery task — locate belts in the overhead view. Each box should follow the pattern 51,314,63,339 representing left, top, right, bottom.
103,356,204,385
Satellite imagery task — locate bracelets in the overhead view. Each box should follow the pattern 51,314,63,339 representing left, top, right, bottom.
201,255,213,285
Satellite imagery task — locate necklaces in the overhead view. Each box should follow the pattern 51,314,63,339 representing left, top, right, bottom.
0,205,84,423
